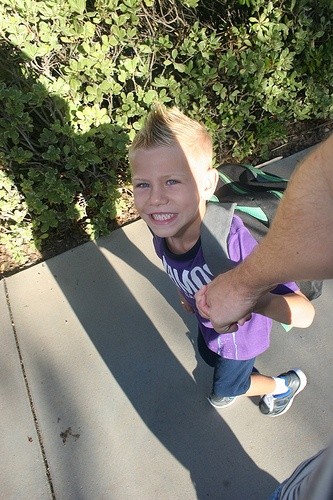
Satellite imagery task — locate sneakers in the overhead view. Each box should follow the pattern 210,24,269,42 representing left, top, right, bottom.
206,365,258,407
260,369,306,417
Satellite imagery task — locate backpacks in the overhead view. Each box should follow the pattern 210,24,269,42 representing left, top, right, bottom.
198,162,322,332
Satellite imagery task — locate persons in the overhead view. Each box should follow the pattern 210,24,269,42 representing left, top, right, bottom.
127,100,315,417
202,133,332,499
